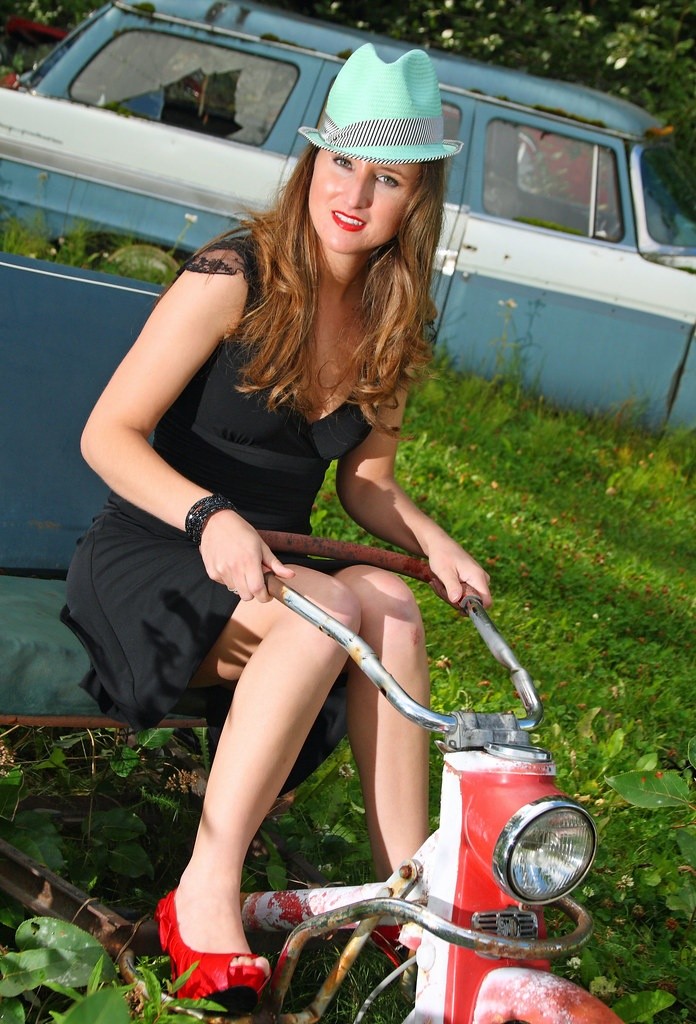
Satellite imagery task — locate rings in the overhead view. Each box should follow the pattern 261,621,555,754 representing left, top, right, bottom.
227,588,237,593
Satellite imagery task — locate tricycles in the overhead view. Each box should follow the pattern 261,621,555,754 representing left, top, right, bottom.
1,251,623,1024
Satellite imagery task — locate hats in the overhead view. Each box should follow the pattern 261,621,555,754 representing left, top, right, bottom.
299,42,465,164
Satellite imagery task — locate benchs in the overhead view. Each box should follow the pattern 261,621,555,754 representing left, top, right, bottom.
1,249,361,734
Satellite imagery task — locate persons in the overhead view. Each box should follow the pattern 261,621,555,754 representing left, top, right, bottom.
76,114,494,1017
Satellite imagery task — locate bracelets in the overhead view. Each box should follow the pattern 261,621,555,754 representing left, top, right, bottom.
184,492,235,546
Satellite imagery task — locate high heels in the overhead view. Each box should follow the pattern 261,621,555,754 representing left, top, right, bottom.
155,892,272,1018
372,925,410,965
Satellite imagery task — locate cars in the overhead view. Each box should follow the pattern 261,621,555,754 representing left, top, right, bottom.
1,0,694,436
